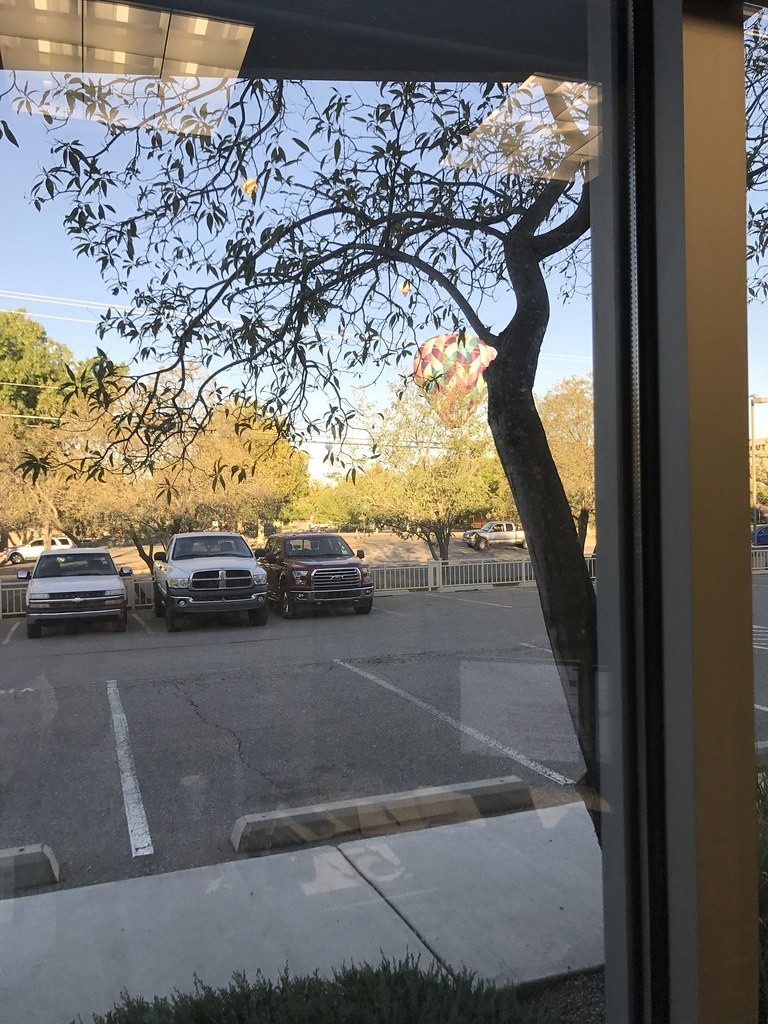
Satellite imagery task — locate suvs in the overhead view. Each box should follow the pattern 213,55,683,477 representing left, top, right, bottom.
257,531,375,615
2,535,74,565
149,531,270,627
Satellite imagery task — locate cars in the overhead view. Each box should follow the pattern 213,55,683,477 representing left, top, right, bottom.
750,525,768,545
17,547,134,632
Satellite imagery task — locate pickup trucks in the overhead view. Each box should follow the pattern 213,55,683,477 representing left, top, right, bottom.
461,520,528,552
